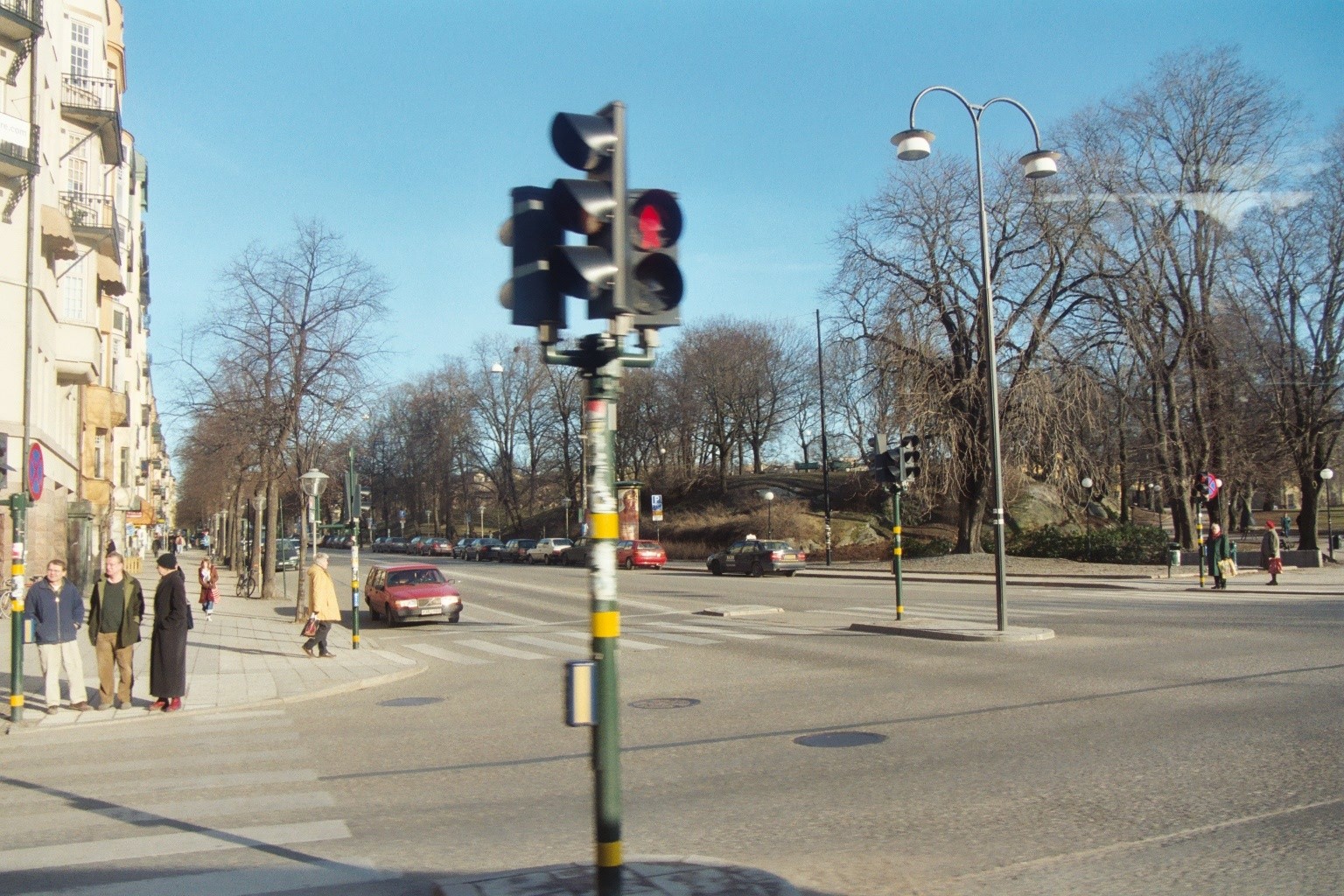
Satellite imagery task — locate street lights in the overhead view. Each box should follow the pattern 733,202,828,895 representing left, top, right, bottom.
479,505,487,538
764,492,775,540
1154,485,1163,531
1321,468,1338,562
251,493,267,592
213,512,220,553
892,84,1063,619
1082,478,1094,562
299,468,331,561
219,509,228,559
425,510,432,538
560,496,572,538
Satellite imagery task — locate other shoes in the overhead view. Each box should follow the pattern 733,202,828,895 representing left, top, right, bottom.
319,651,337,657
47,704,58,715
207,615,212,622
119,701,132,710
69,701,94,712
147,698,169,711
1266,580,1278,586
97,701,114,710
302,645,317,658
165,698,181,712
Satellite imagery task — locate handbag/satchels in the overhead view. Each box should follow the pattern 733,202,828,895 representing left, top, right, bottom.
300,618,319,636
1217,558,1237,578
187,601,193,629
1268,557,1283,574
211,587,221,603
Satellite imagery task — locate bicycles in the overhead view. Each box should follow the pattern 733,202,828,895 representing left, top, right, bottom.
236,566,256,596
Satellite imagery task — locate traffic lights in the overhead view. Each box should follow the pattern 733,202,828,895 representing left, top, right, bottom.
542,100,627,321
868,433,885,483
354,483,373,520
882,448,905,484
900,433,922,480
627,189,683,330
495,186,564,328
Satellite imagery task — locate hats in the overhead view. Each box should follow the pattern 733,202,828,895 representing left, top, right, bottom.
157,553,177,570
1266,520,1274,528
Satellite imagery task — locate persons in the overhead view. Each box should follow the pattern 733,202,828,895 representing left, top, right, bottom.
1205,522,1230,589
197,559,219,622
1260,520,1282,586
301,552,341,660
149,528,211,559
104,539,116,560
145,554,189,713
23,559,92,716
1281,511,1292,538
86,551,145,712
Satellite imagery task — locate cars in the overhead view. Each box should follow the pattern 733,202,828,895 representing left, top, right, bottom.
559,535,590,568
296,530,573,565
613,539,667,570
367,564,465,625
706,533,807,579
271,540,300,571
